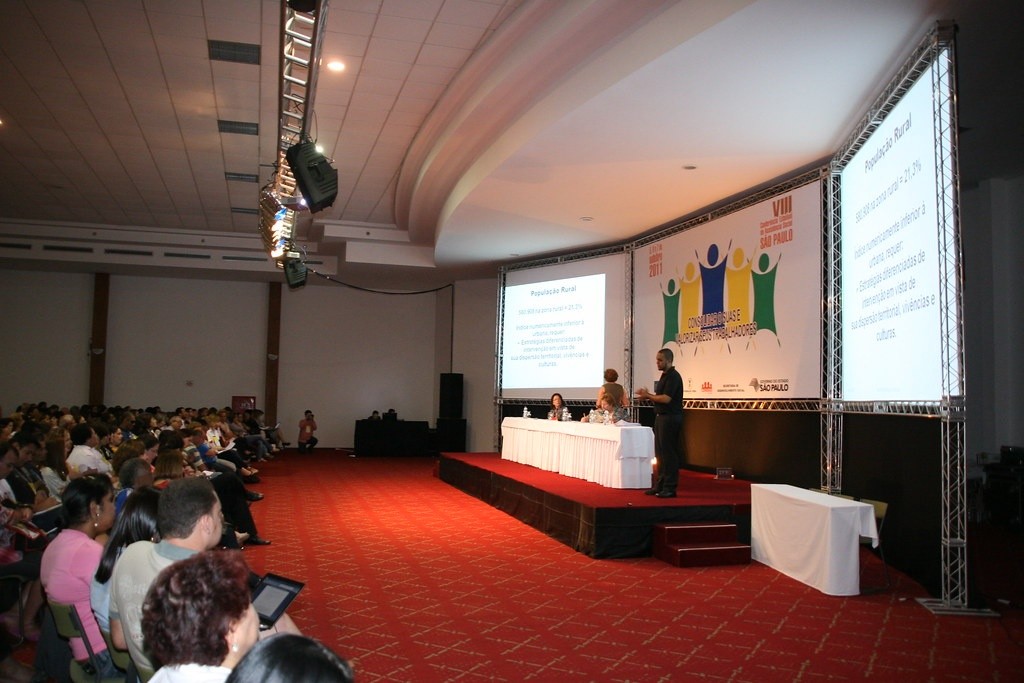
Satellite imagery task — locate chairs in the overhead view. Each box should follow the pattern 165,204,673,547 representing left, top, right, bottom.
859,498,891,574
0,570,142,683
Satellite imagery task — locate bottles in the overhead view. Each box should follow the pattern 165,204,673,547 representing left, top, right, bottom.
588,409,612,426
523,407,528,418
562,407,572,421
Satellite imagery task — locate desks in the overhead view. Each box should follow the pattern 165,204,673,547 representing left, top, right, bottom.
749,482,879,596
500,417,655,489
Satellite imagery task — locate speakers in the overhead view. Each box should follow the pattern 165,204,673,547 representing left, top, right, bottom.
283,258,307,289
436,373,467,456
286,142,338,213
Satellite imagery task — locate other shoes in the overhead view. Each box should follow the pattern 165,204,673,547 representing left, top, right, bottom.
264,453,274,460
258,457,268,463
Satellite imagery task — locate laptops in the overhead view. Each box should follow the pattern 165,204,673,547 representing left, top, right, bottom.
252,572,305,631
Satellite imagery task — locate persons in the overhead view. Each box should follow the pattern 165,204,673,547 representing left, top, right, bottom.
297,410,319,454
635,348,683,498
368,408,395,420
0,401,291,642
581,393,632,423
40,478,223,683
144,553,260,683
550,393,567,420
597,369,630,409
225,633,353,683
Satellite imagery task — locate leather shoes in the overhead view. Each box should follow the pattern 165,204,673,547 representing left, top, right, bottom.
645,489,658,495
654,489,675,498
244,536,271,545
245,489,264,501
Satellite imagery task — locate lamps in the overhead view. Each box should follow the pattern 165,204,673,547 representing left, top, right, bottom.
257,181,293,257
